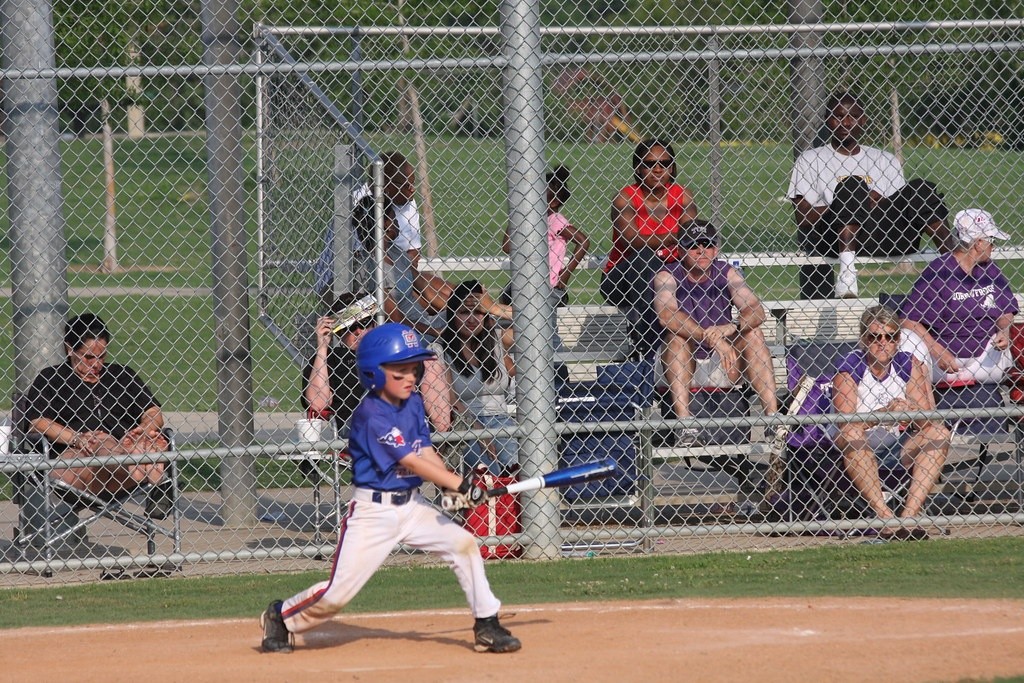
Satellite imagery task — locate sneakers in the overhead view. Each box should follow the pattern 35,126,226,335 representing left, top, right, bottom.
473,612,521,653
260,599,295,653
834,267,858,298
674,414,704,446
765,412,784,443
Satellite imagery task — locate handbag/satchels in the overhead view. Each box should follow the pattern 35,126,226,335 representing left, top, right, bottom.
463,463,521,558
775,375,836,448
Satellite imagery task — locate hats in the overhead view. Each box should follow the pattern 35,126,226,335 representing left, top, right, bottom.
678,220,716,246
952,208,1011,243
385,355,436,364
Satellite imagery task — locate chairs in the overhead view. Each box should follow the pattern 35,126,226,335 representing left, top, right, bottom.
879,291,1023,513
782,338,918,520
19,428,179,573
550,358,655,509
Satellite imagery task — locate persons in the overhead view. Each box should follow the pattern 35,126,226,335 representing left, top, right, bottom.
651,220,787,447
23,313,167,492
260,324,523,653
300,291,474,468
786,92,955,299
313,152,455,315
420,280,519,474
600,139,698,361
499,165,589,307
899,208,1019,426
352,195,450,346
826,305,951,539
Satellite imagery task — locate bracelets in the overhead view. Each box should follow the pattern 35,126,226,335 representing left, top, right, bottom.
73,432,83,448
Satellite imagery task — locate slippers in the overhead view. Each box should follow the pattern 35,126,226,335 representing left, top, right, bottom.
100,565,132,581
133,564,171,578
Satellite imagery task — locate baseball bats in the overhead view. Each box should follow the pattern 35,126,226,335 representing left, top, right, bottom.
442,458,618,511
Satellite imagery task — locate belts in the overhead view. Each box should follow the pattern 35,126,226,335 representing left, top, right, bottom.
373,488,420,506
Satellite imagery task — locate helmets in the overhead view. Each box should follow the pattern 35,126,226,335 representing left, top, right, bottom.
355,323,435,392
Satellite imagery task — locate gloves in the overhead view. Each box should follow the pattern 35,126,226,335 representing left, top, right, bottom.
441,488,475,511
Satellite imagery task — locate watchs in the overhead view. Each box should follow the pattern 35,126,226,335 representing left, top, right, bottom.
732,320,741,335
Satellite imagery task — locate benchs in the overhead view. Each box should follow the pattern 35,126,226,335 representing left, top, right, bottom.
419,247,1023,459
307,408,463,559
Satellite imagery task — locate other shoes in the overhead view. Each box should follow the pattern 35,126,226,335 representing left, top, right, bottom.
877,526,929,540
432,413,471,471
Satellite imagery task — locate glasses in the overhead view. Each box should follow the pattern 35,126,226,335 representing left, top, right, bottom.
867,332,898,343
981,237,997,245
683,241,715,250
639,159,672,169
341,314,377,333
